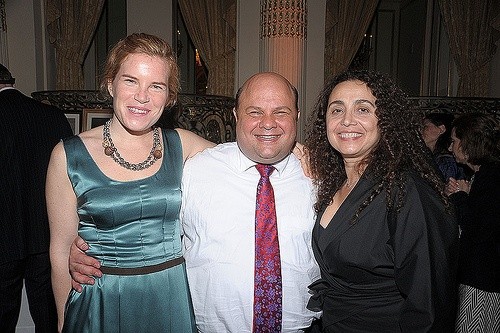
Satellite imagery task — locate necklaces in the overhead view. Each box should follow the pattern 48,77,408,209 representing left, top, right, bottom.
103,120,162,170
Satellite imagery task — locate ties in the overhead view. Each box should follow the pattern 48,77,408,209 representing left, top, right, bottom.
252,164,282,333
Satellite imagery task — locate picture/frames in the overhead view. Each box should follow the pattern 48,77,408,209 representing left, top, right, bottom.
63,109,82,135
81,108,113,133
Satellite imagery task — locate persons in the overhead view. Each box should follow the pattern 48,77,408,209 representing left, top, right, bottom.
69,72,321,333
445,112,499,333
0,64,75,333
305,68,459,333
419,114,458,179
46,33,314,333
